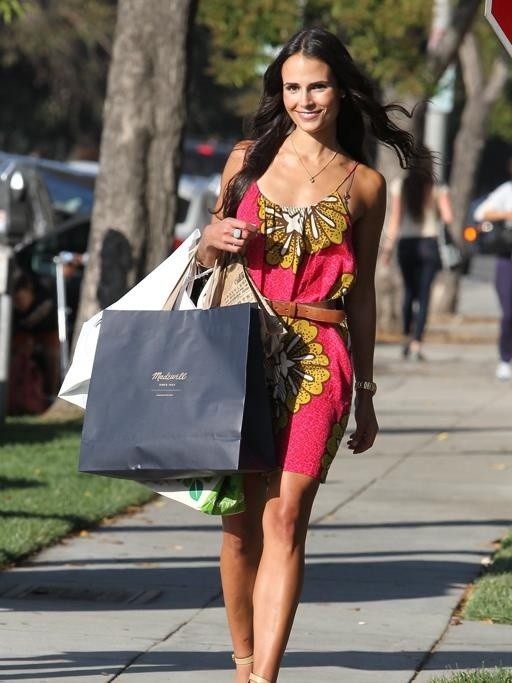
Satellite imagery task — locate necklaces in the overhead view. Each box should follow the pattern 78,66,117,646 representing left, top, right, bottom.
290,133,340,183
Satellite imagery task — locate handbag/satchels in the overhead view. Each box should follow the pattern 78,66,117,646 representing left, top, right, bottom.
58,229,285,516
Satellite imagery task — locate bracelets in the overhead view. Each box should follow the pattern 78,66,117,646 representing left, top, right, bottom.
196,257,214,270
355,380,376,395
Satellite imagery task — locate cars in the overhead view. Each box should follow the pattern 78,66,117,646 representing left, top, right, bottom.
0,142,232,422
461,195,511,255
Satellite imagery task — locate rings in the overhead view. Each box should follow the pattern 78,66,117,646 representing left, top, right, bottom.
234,227,242,239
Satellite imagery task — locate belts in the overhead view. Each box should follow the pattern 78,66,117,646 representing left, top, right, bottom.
265,298,348,324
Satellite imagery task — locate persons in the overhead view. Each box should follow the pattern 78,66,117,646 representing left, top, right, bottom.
196,28,441,683
473,180,512,380
381,148,460,360
